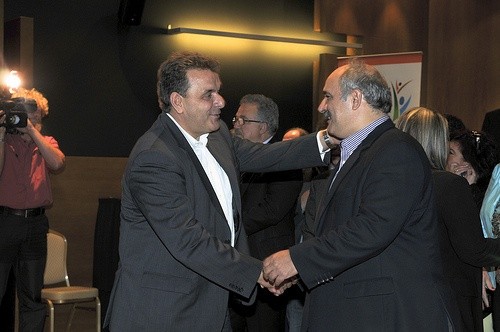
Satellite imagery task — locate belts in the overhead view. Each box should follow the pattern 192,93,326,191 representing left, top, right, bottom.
0,205,46,218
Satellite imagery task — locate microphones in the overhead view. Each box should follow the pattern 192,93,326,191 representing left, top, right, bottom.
3,100,15,108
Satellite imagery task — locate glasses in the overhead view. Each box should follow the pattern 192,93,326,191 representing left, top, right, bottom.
232,117,268,126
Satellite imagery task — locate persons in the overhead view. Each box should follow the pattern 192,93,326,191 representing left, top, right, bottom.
263,62,464,332
233,95,329,332
0,88,65,332
397,107,500,332
102,51,341,332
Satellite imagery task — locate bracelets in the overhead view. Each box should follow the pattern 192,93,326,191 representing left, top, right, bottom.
323,131,335,149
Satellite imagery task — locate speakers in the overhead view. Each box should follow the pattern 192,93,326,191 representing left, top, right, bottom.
117,0,146,27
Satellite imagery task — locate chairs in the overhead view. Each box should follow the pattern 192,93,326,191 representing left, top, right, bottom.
15,229,101,332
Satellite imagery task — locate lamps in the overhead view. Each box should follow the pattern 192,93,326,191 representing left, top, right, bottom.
167,23,363,49
